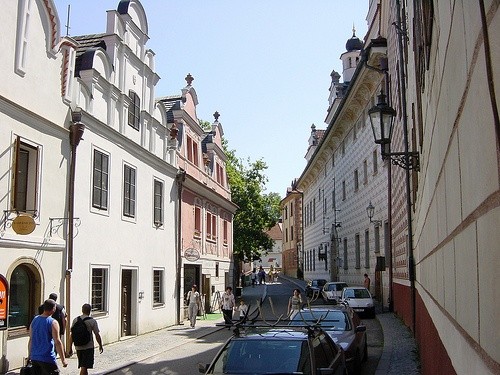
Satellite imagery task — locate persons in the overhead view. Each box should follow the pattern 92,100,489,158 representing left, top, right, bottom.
268,261,279,282
287,288,303,316
238,299,248,325
222,287,235,329
251,266,266,287
27,299,68,375
364,274,370,291
68,303,103,375
37,293,67,356
186,285,200,328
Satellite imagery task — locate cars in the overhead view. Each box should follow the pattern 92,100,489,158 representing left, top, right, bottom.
339,287,375,318
305,279,328,298
322,282,348,304
199,319,345,375
288,305,368,373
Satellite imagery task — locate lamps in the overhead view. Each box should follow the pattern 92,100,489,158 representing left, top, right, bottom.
368,89,420,172
335,221,342,232
320,242,329,250
139,291,144,300
330,238,341,247
366,200,382,226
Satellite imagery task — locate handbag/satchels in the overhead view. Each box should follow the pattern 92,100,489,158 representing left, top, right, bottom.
186,292,191,306
20,358,32,375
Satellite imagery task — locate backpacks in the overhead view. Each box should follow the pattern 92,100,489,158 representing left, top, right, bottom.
71,316,93,346
